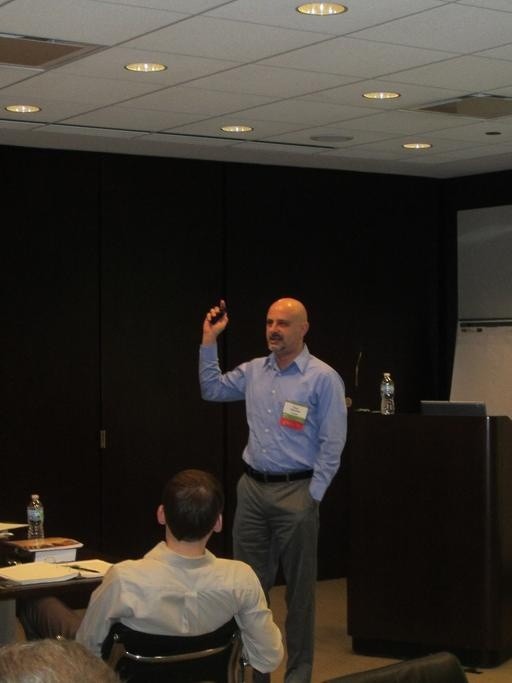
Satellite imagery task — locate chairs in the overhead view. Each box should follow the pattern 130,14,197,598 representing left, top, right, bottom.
326,651,466,681
102,615,249,682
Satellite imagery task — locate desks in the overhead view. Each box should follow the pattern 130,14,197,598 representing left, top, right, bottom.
0,522,118,642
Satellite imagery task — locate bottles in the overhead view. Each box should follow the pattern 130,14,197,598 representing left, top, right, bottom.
27,494,45,538
379,372,395,416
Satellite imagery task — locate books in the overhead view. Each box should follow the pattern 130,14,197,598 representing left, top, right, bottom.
1,557,117,586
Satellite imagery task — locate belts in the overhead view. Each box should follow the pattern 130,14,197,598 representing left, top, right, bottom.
239,457,315,484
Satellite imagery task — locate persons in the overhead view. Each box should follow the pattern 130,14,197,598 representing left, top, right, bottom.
194,294,350,682
15,466,286,674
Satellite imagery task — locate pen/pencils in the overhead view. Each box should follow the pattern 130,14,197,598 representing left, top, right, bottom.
68,566,100,573
